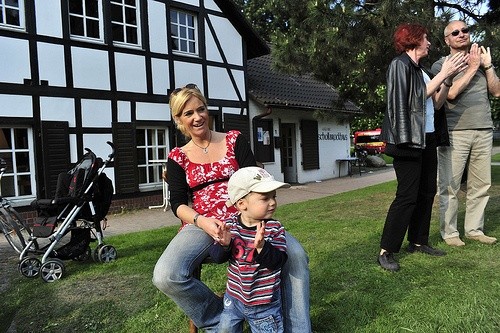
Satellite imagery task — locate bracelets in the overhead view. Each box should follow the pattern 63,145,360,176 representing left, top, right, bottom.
443,82,452,87
484,64,493,71
193,213,200,226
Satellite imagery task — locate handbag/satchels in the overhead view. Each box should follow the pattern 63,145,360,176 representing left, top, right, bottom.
80,173,113,223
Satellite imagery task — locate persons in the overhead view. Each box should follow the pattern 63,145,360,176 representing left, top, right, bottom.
377,23,470,271
214,167,288,333
430,20,500,246
152,83,312,333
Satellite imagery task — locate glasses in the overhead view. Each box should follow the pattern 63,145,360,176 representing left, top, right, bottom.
170,83,199,102
446,27,470,38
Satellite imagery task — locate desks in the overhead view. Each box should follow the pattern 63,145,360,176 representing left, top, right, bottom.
336,158,361,179
149,159,170,212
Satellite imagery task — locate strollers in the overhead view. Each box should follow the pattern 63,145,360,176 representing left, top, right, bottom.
18,140,118,283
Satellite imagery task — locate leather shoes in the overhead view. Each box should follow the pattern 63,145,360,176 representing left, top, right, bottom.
407,242,447,256
376,250,400,270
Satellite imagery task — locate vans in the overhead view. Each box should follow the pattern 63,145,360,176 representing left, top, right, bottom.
355,128,388,153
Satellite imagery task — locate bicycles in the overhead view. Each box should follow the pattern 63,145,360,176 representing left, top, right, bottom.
0,157,40,258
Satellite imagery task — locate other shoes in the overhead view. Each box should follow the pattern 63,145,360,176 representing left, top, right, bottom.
466,234,497,244
445,236,465,246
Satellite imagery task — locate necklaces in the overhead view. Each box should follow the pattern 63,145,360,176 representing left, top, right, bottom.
192,130,212,153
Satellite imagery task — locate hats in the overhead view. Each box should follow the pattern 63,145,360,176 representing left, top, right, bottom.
228,167,290,203
